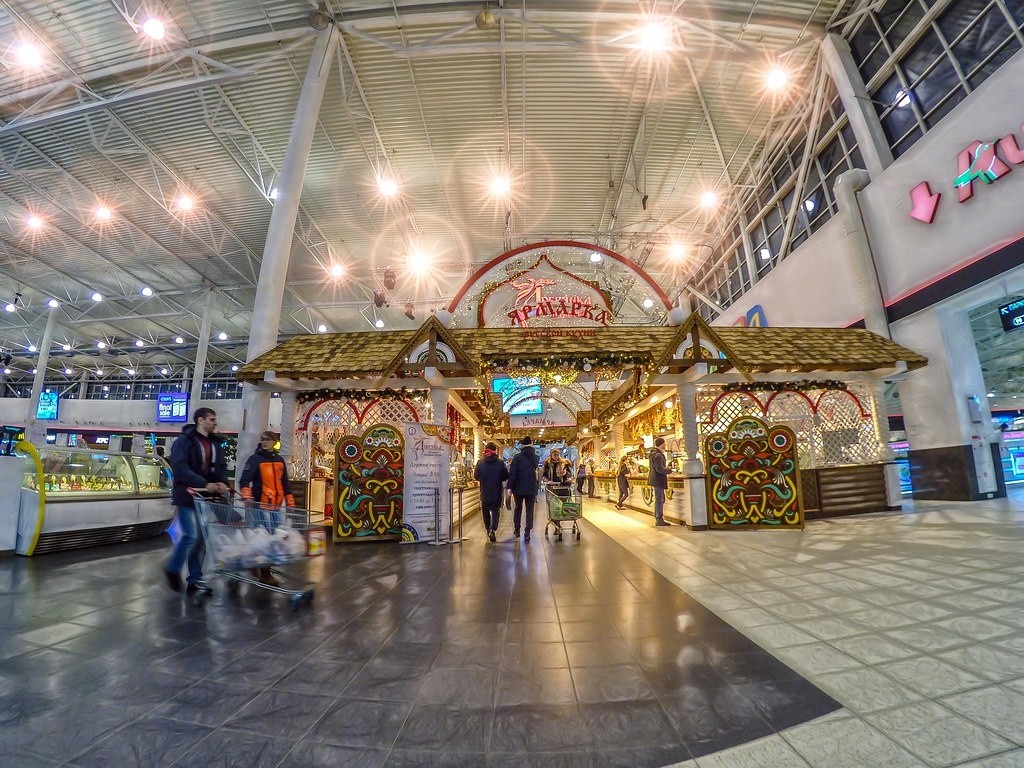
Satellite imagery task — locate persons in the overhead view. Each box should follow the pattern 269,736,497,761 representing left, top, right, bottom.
160,408,297,593
474,436,671,543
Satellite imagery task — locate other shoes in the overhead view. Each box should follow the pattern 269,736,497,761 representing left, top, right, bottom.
620,507,627,510
524,533,530,541
615,504,619,510
187,581,212,594
514,530,520,537
488,529,496,542
163,564,183,592
553,528,558,535
655,520,670,526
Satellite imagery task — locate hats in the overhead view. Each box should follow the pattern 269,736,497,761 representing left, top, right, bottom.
655,437,665,446
521,436,531,445
485,442,496,452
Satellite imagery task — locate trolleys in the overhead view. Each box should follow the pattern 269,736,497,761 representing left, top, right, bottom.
188,485,326,615
542,481,583,543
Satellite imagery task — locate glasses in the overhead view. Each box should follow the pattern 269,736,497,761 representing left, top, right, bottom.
260,437,273,442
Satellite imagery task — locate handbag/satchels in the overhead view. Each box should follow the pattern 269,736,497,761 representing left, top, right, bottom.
215,523,308,571
